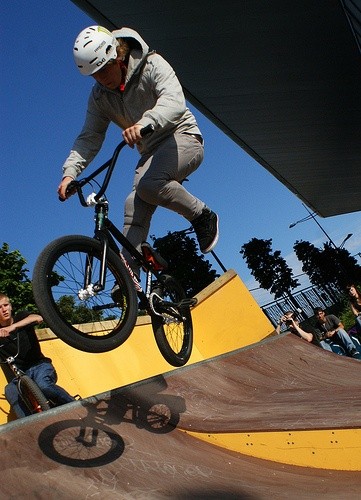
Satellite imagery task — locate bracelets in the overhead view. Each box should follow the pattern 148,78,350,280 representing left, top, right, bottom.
334,330,337,333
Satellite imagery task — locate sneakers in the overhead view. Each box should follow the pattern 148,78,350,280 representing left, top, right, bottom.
190,203,219,254
110,267,141,305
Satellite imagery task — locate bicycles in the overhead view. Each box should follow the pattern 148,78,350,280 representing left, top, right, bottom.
0,320,51,417
31,124,197,368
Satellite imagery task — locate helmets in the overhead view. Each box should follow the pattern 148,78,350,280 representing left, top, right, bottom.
73,25,120,76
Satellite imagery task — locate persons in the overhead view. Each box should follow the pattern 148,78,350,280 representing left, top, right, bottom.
57,26,219,300
0,293,78,420
275,285,361,359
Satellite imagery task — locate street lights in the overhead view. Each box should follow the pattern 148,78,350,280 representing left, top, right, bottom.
337,232,353,250
288,204,339,250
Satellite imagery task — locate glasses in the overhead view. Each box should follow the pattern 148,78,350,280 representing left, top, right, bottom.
316,312,324,316
